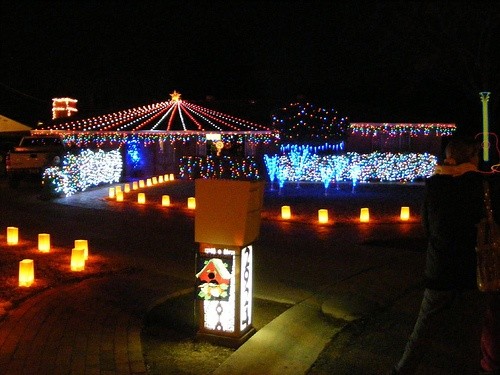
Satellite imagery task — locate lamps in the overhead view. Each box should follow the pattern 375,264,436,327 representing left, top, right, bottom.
70,247,85,272
137,193,146,205
116,191,123,201
108,173,174,199
280,206,291,220
187,197,197,209
74,239,88,261
162,195,170,206
38,232,50,254
18,258,36,288
6,227,19,246
399,206,410,222
318,209,329,223
359,208,370,223
